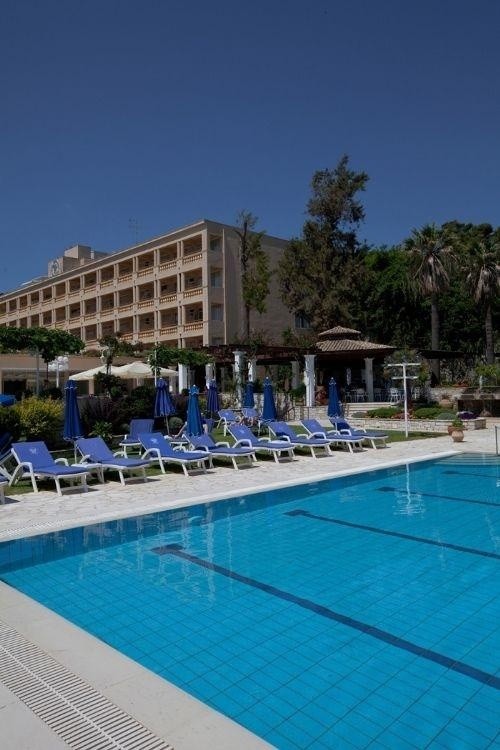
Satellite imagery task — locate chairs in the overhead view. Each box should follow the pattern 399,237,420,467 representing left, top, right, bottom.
343,387,420,404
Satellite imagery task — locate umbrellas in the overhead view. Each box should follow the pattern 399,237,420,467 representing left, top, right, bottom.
327,377,344,446
69,360,277,437
63,379,86,464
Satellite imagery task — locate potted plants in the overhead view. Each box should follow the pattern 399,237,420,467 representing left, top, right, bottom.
448,416,468,435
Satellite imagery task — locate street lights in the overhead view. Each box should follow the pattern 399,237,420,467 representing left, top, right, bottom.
48,356,69,388
147,348,157,390
100,348,119,375
